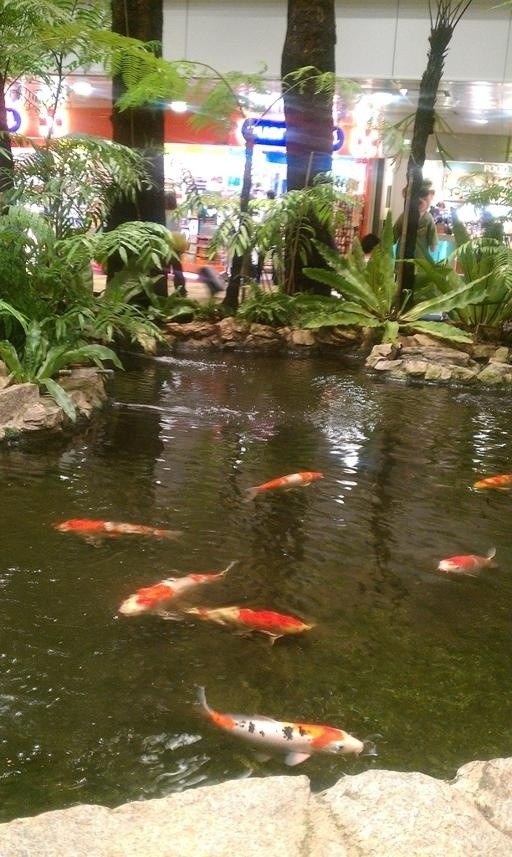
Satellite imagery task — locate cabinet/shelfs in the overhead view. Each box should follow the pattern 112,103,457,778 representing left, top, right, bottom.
196,216,218,258
335,195,363,253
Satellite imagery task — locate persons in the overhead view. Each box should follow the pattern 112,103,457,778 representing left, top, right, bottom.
428,202,445,224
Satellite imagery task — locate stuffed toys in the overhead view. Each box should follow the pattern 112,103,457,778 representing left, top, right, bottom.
390,179,437,281
